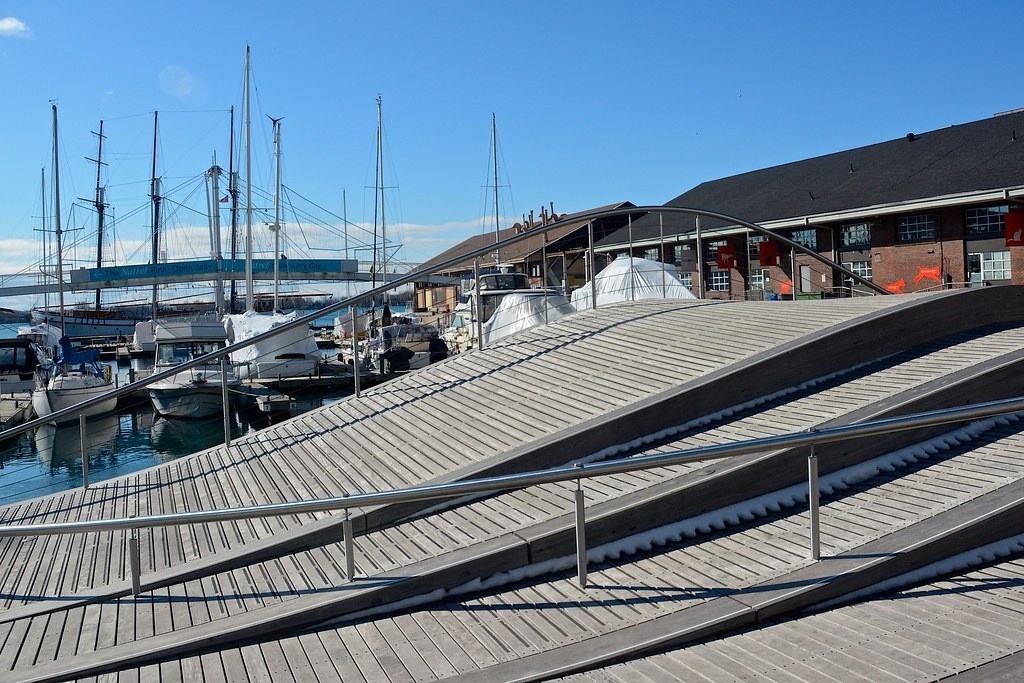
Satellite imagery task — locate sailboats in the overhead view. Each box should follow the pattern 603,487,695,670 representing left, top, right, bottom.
31,99,119,428
0,45,578,394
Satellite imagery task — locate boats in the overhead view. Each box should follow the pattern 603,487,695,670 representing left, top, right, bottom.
145,321,242,419
